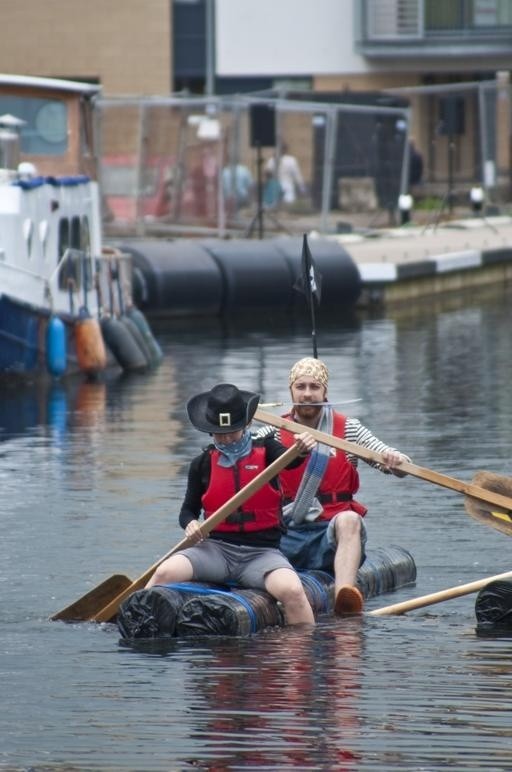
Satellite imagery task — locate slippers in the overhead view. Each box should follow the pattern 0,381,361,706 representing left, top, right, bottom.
335,585,362,617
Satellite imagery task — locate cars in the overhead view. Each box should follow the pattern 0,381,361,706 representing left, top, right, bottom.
92,152,203,229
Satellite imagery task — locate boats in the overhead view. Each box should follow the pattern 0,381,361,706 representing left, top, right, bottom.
115,536,423,649
2,69,170,403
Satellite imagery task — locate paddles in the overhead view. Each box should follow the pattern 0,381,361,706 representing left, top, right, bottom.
49,444,302,623
254,409,512,533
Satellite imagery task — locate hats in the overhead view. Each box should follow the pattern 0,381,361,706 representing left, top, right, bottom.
187,384,260,434
288,358,329,394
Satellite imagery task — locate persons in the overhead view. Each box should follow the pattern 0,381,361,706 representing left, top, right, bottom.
309,630,372,772
257,166,286,212
244,352,416,619
408,135,425,183
175,621,319,772
220,152,256,209
263,140,308,207
141,384,316,629
